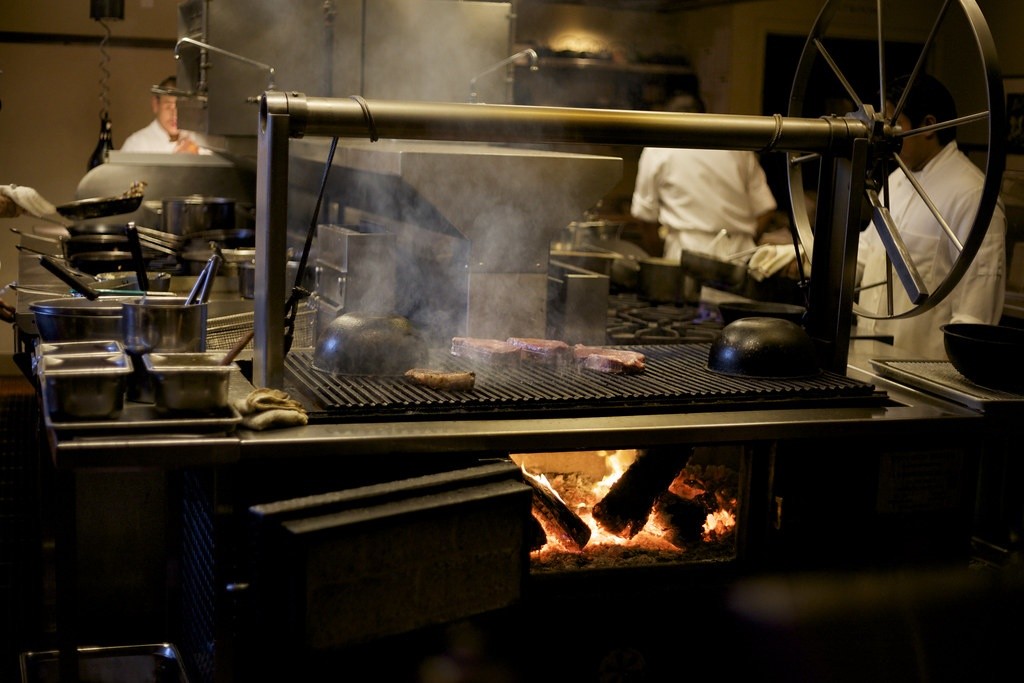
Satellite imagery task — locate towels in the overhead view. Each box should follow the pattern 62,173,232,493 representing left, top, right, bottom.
234,387,309,431
747,244,804,283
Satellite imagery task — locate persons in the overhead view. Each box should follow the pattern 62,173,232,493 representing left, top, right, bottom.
0,183,60,219
630,95,775,305
120,76,213,155
855,73,1006,359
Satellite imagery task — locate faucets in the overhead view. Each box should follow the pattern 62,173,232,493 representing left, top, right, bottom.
174,35,277,104
469,48,540,102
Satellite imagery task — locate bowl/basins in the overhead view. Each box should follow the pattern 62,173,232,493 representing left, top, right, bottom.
718,302,807,327
34,340,242,422
307,311,429,378
940,323,1024,396
703,316,816,380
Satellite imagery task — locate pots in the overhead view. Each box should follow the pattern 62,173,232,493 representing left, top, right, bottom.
0,181,309,358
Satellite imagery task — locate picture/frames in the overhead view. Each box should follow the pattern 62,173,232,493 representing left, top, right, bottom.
1003,74,1024,171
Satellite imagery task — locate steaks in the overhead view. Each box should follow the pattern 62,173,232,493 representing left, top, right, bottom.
404,368,474,390
448,336,647,373
